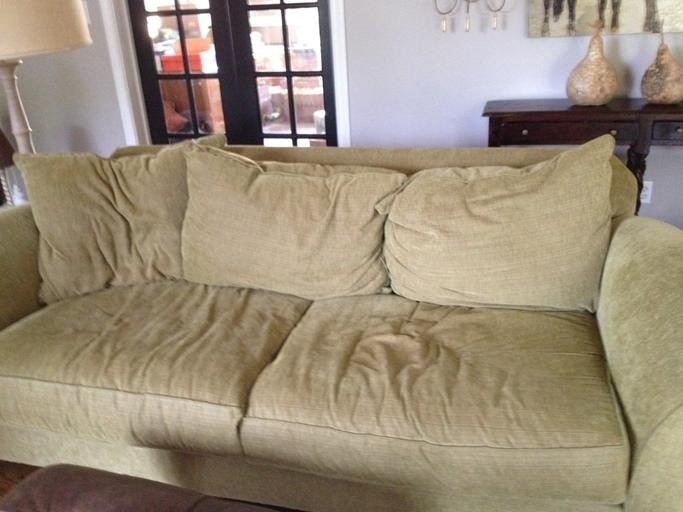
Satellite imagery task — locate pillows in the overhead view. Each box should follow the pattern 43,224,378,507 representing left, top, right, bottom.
178,140,409,303
12,136,230,306
373,136,616,318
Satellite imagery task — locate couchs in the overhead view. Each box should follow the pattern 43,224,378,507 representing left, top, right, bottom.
0,134,683,510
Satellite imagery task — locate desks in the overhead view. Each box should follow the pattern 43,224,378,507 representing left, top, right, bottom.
481,99,683,218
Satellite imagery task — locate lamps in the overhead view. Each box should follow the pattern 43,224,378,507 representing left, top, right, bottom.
0,1,95,153
